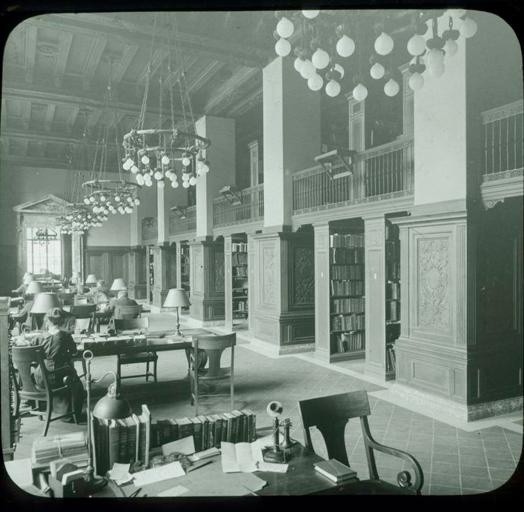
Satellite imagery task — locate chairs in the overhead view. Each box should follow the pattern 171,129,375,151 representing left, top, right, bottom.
9,269,151,332
8,342,80,438
296,389,424,494
188,330,237,418
112,313,162,392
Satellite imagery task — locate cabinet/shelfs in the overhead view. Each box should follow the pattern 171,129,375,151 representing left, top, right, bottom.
176,244,191,317
360,214,400,385
312,222,364,365
222,236,248,332
146,246,155,305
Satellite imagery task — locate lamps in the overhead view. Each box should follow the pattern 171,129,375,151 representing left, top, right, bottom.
120,12,213,191
64,126,103,231
76,68,142,215
270,8,477,107
77,348,135,492
160,286,192,337
54,146,88,237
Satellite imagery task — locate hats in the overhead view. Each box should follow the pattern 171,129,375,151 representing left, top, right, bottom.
45,307,64,319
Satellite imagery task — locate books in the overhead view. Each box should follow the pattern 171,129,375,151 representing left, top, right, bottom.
180,245,190,309
148,247,154,304
231,242,248,319
330,222,400,378
311,457,357,483
28,407,258,500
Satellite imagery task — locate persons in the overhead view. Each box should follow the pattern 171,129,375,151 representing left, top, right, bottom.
108,289,138,331
41,304,74,334
86,279,107,305
29,307,83,425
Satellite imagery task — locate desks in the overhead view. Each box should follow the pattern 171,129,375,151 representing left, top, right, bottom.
4,425,365,496
9,328,228,406
